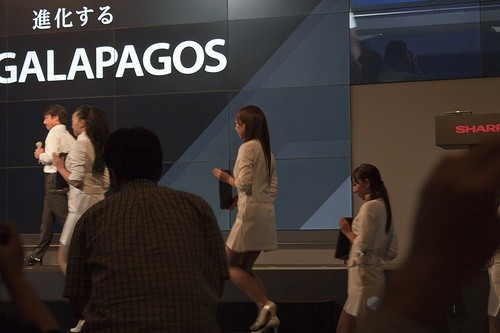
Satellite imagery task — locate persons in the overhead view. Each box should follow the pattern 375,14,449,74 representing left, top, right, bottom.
52,104,110,332
0,225,60,333
212,104,281,333
23,103,78,266
350,39,427,84
61,126,231,333
488,203,500,333
336,163,399,333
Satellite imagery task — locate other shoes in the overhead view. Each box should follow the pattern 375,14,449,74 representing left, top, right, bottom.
23,256,42,266
70,320,84,332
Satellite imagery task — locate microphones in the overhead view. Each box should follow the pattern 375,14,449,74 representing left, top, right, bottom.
36,141,43,164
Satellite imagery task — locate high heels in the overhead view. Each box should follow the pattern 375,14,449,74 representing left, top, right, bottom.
251,316,279,333
249,301,278,332
